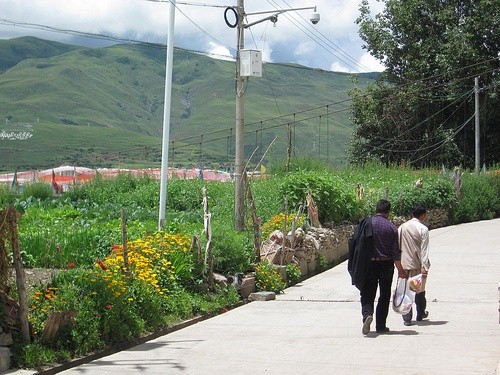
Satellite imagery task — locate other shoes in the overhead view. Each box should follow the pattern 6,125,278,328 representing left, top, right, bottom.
375,327,390,333
361,316,373,335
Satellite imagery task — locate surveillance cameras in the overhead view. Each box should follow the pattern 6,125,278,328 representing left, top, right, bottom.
310,12,319,24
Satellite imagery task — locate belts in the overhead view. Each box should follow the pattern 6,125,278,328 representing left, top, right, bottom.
367,257,393,262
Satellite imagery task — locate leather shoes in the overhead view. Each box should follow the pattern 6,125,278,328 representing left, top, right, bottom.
415,311,429,321
403,321,411,326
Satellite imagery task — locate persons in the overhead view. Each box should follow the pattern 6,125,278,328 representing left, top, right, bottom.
397,205,431,326
348,199,407,335
